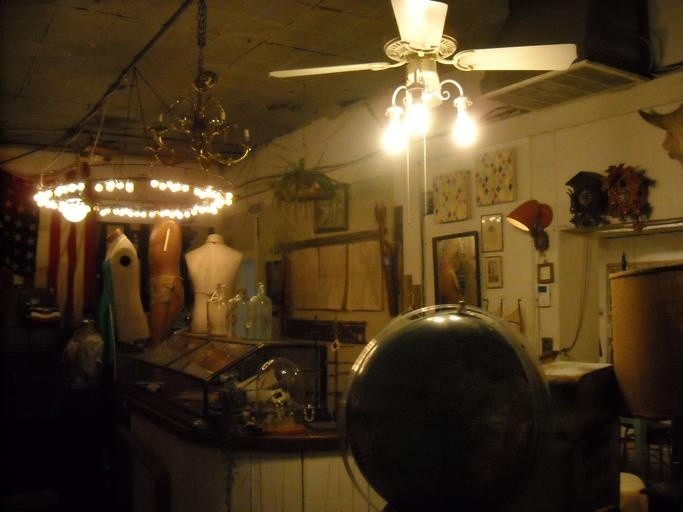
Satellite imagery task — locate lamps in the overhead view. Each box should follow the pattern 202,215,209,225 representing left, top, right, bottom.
143,0,253,175
372,57,485,159
505,196,553,258
29,63,235,224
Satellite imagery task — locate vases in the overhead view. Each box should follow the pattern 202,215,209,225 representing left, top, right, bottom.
205,276,274,342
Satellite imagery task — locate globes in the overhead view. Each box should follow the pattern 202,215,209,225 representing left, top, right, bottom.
338,303,553,512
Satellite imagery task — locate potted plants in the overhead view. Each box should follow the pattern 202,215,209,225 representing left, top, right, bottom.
262,142,350,219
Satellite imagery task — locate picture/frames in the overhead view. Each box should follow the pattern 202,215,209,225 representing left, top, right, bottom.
430,211,503,314
310,181,349,234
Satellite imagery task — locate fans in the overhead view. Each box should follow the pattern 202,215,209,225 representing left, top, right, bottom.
267,0,582,80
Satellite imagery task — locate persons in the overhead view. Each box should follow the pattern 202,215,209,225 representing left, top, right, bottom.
440,245,462,304
445,237,469,295
105,228,150,343
185,234,243,336
147,217,184,348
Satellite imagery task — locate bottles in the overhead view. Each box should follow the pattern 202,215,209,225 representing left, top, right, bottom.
60,317,105,389
245,282,273,340
206,282,232,338
227,288,248,339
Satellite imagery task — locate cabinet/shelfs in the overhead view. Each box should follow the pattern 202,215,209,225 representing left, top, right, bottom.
119,328,328,436
